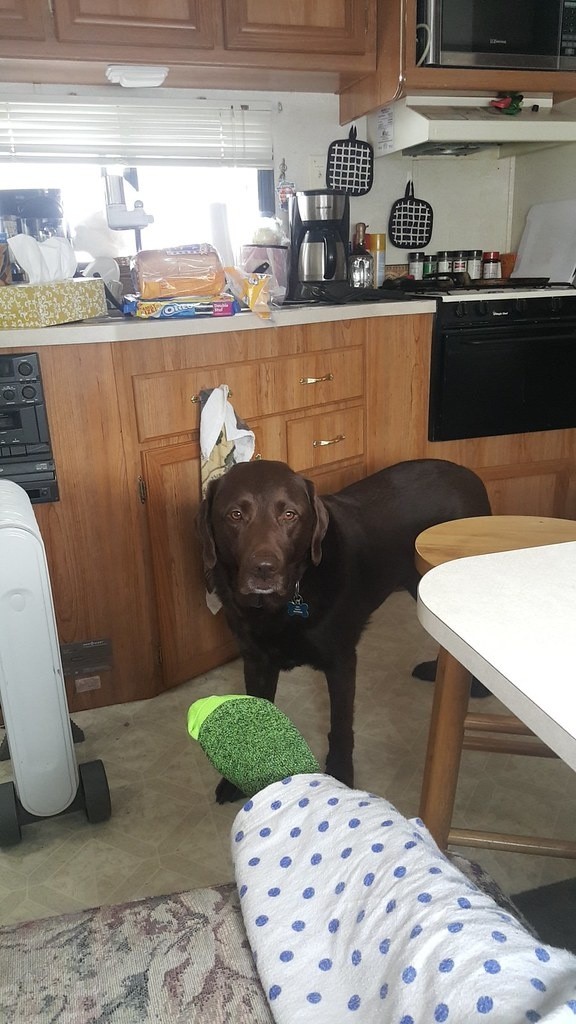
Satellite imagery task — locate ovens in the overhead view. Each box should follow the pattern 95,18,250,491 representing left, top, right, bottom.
427,322,576,442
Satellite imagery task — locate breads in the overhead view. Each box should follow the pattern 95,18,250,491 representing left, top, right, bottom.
133,242,224,302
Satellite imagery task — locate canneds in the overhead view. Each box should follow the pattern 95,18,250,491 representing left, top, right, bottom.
407,249,501,281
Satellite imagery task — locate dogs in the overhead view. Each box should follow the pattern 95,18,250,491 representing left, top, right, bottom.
193,457,494,806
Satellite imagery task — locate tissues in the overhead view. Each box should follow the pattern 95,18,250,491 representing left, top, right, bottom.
0,232,109,331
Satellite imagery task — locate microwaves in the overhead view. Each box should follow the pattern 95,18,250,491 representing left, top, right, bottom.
414,0,576,74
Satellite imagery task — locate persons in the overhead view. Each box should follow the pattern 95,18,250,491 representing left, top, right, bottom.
180,684,574,1024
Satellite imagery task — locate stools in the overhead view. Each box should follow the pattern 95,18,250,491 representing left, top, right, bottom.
415,515,576,860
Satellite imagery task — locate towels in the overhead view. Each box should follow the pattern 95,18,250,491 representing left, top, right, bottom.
198,382,256,618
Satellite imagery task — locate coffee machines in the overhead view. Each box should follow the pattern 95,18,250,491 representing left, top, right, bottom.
286,188,354,304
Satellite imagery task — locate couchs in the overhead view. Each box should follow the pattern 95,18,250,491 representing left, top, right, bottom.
1,852,537,1024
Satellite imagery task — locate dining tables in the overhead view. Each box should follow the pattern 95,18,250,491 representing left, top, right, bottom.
416,541,576,775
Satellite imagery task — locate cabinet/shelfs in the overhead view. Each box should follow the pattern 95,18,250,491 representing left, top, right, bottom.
52,0,215,53
0,1,47,44
223,0,366,57
112,317,369,689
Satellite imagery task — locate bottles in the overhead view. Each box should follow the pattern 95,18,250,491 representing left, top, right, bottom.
347,222,375,290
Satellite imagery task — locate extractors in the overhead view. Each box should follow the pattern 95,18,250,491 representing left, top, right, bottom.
364,92,576,157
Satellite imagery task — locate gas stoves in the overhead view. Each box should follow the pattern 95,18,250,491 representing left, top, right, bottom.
373,275,576,327
